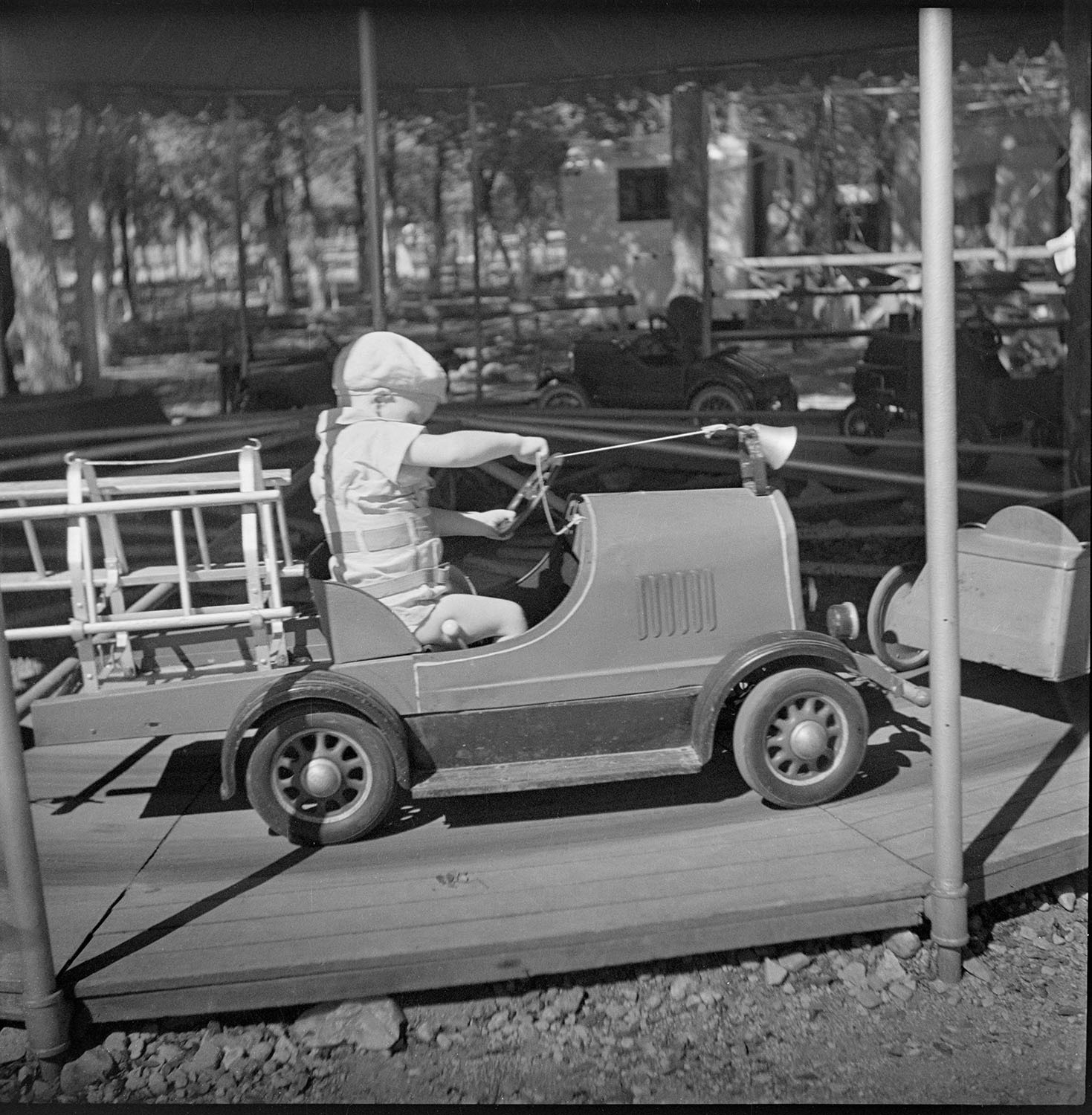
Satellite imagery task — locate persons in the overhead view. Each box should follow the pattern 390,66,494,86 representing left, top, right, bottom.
309,331,549,644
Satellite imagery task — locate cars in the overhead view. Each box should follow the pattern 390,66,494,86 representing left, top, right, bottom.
530,329,798,445
839,283,1061,473
868,496,1091,686
222,430,929,843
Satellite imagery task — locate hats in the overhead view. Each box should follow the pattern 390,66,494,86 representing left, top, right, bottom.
332,332,447,405
